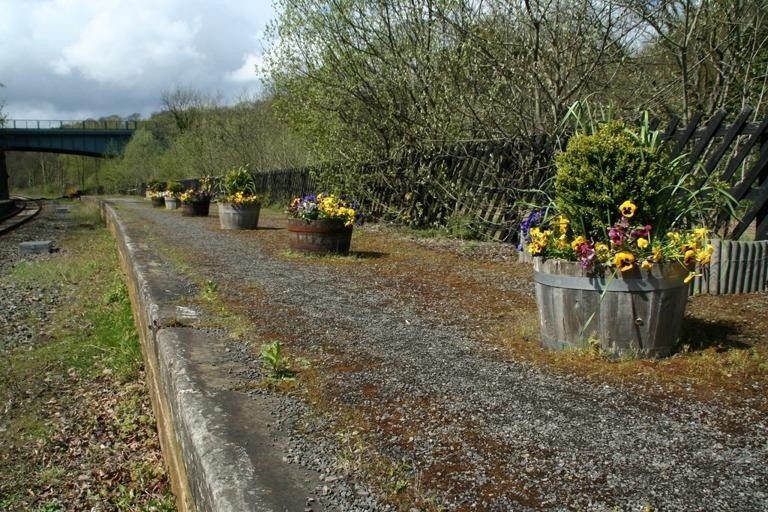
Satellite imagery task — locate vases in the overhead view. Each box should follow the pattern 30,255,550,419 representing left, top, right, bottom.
218,203,260,229
289,218,352,255
153,198,163,207
534,254,691,357
166,198,180,209
183,201,210,217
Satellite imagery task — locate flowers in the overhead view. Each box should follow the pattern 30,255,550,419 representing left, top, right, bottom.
518,92,744,336
212,167,259,205
284,193,363,227
162,179,185,197
147,179,162,198
179,175,213,203
